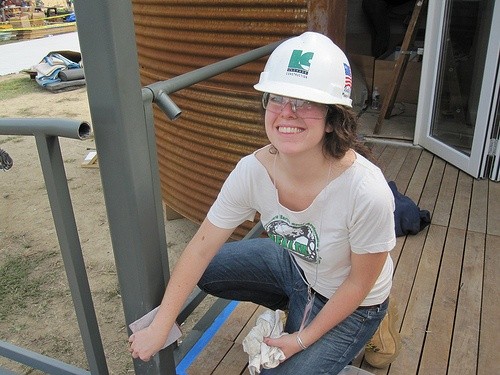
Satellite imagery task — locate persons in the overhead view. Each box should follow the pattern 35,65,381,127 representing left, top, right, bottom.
128,31,400,375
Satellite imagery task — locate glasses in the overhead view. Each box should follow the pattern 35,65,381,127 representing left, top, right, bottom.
260,91,333,120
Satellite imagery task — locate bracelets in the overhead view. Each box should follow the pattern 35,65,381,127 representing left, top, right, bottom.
296,330,307,350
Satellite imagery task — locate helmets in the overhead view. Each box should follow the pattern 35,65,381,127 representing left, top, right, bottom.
253,31,353,111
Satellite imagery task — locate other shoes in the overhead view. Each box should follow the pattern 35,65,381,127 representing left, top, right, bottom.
364,294,401,370
